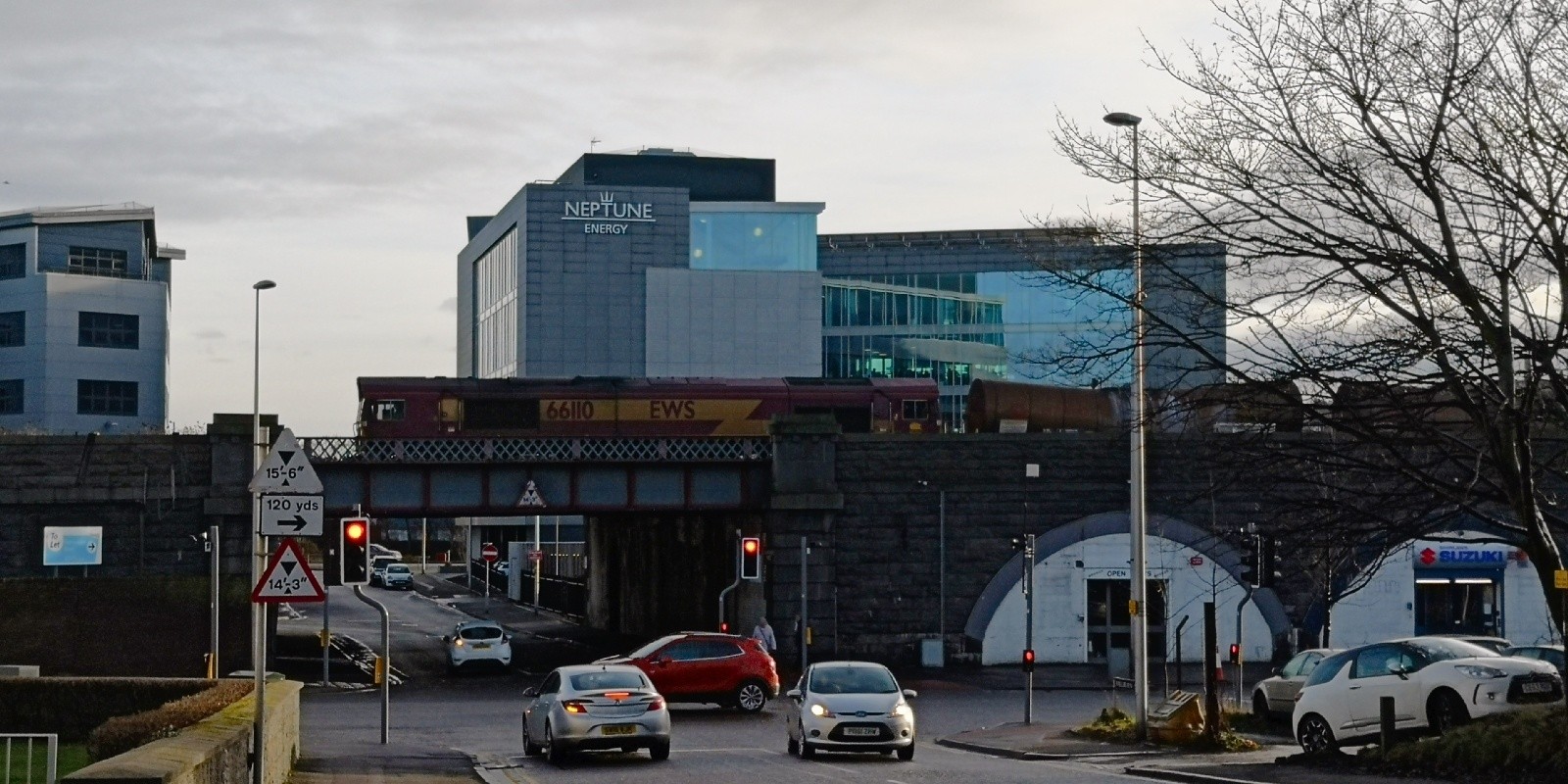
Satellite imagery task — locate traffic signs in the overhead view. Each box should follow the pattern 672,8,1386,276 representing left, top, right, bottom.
259,494,325,535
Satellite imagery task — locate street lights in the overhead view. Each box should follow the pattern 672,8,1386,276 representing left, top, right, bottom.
1102,111,1149,737
251,278,277,784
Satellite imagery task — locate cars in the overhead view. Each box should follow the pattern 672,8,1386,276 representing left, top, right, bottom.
442,619,511,675
369,543,414,591
522,664,670,770
1250,635,1567,766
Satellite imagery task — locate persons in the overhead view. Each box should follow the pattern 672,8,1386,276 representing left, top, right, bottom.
792,612,803,666
752,615,777,653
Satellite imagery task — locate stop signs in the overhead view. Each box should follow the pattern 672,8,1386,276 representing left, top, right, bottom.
483,545,497,561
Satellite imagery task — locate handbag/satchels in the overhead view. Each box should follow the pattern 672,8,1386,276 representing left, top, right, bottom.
767,645,776,655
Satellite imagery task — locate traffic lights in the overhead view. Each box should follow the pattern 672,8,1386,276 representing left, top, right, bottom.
1229,644,1238,664
340,518,371,586
1241,534,1260,587
721,623,730,635
1022,649,1036,673
742,538,760,579
1263,538,1281,589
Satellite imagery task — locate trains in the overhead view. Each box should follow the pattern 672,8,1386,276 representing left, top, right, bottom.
355,374,937,456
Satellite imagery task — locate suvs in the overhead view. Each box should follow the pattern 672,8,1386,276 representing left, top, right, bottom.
591,631,780,715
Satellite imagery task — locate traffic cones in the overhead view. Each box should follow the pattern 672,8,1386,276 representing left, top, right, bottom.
1216,647,1228,685
